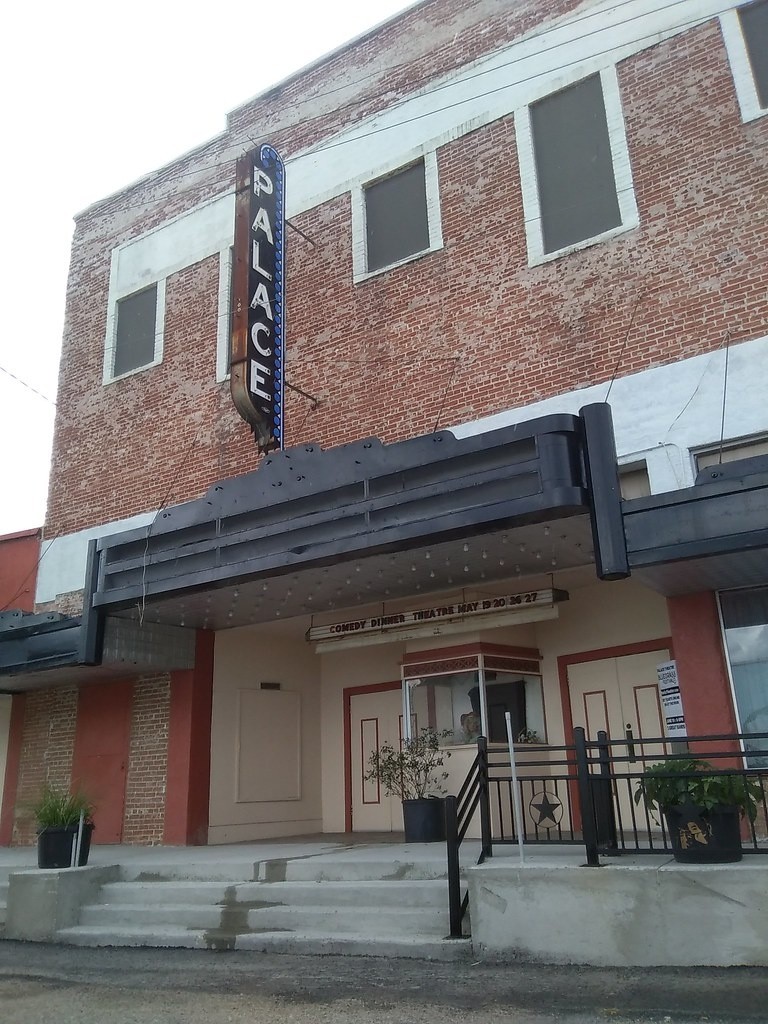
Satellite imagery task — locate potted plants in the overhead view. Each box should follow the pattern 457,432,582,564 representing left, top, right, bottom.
362,725,456,845
28,779,96,869
637,751,765,866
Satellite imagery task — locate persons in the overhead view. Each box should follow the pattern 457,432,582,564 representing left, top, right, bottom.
464,712,481,744
455,713,472,744
